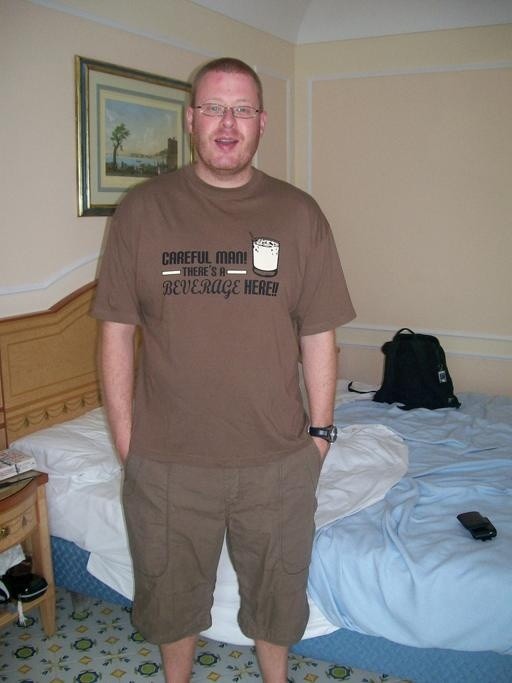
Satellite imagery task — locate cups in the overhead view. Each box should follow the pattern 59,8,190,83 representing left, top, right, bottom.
252,236,280,276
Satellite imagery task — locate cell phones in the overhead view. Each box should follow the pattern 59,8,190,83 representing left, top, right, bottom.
469,517,497,540
456,511,489,530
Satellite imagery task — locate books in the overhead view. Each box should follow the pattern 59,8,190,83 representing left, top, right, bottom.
0,445,38,481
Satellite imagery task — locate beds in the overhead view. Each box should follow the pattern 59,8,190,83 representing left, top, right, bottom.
2,250,511,681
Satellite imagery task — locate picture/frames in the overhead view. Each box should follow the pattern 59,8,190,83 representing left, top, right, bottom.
74,52,196,218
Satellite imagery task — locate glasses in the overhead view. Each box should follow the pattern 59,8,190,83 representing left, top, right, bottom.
194,103,263,119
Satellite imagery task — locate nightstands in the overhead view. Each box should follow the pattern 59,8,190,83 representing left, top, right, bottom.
1,468,58,641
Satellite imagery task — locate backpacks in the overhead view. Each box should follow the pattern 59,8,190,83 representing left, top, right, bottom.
373,327,461,411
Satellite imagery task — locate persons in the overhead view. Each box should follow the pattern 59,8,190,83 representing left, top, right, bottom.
89,55,361,682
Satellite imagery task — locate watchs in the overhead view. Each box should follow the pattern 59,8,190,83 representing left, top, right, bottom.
308,421,339,444
